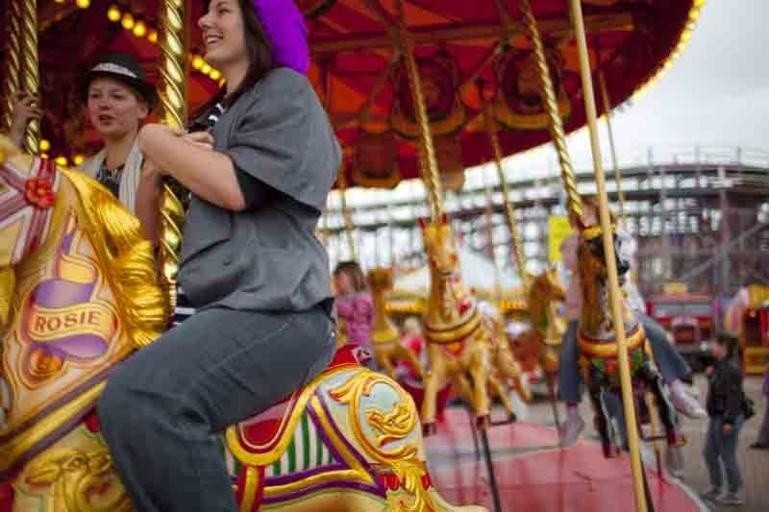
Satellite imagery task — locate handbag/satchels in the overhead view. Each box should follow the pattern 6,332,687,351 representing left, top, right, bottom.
741,395,760,419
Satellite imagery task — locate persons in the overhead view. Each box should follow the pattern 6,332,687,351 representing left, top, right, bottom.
328,260,374,367
7,53,157,253
702,333,746,505
98,1,342,512
749,334,769,448
559,196,707,447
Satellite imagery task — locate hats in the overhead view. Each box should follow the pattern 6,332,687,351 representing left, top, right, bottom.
255,0,310,72
77,53,157,114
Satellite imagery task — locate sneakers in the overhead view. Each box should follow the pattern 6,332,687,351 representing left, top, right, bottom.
559,415,585,447
703,487,743,506
672,391,705,419
